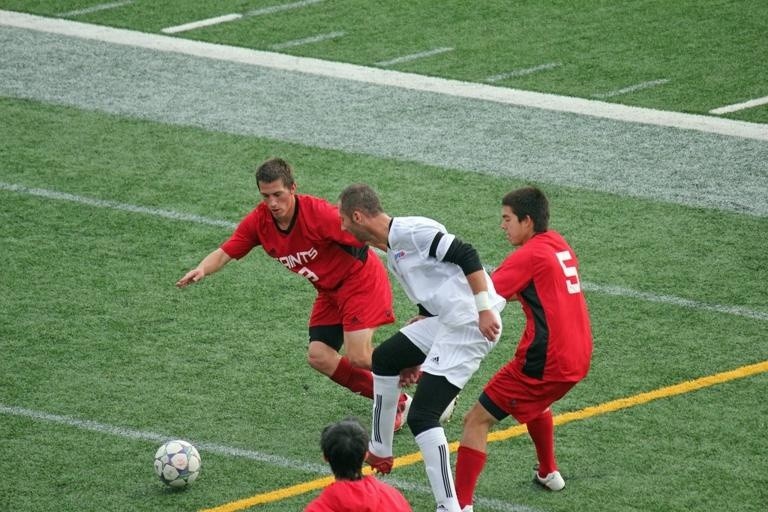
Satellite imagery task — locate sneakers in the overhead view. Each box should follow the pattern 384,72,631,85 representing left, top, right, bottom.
533,464,566,492
394,393,413,433
364,450,393,475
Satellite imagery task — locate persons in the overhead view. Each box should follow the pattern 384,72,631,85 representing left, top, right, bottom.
304,419,410,512
337,184,508,512
454,188,593,512
176,156,458,432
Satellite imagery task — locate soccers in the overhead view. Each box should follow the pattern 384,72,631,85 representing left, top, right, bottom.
153,440,201,490
